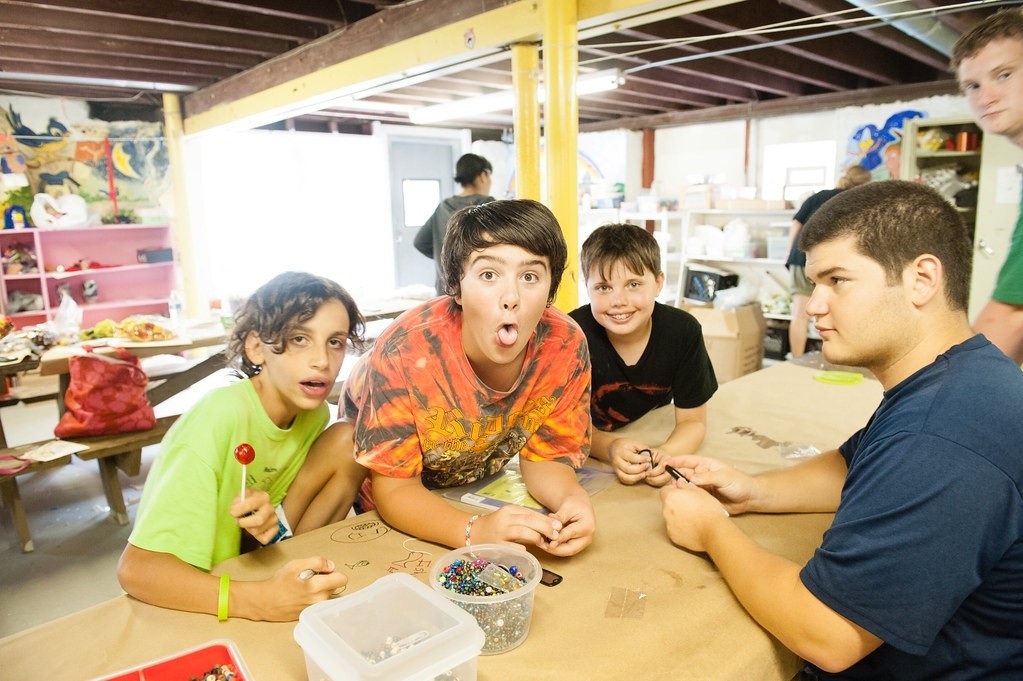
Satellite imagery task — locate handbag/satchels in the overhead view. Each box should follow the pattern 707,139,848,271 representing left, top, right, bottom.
55,345,157,438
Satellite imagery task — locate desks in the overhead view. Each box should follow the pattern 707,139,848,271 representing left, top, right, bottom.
0,352,883,681
40,283,431,423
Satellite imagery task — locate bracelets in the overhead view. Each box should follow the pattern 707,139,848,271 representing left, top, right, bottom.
465,513,491,561
216,574,229,621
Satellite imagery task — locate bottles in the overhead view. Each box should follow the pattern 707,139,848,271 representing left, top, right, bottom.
169,289,187,335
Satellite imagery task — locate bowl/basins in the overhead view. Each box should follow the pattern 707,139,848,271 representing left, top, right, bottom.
430,541,543,656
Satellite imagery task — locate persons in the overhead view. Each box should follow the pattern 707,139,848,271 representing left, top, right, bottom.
415,153,495,296
953,4,1023,368
565,224,718,486
336,199,596,559
785,166,871,359
660,179,1023,681
116,270,368,622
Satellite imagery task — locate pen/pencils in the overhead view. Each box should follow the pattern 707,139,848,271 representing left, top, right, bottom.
665,465,731,518
301,569,316,582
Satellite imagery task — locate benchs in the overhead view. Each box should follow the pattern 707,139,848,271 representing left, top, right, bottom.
0,414,182,552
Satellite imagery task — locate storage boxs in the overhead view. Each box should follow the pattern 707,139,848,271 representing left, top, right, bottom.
292,570,485,681
686,303,767,379
681,183,794,208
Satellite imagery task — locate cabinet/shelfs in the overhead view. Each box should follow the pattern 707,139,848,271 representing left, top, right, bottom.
900,116,981,215
579,207,794,320
2,224,179,332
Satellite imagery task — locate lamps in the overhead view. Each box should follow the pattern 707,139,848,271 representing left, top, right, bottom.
407,67,626,127
